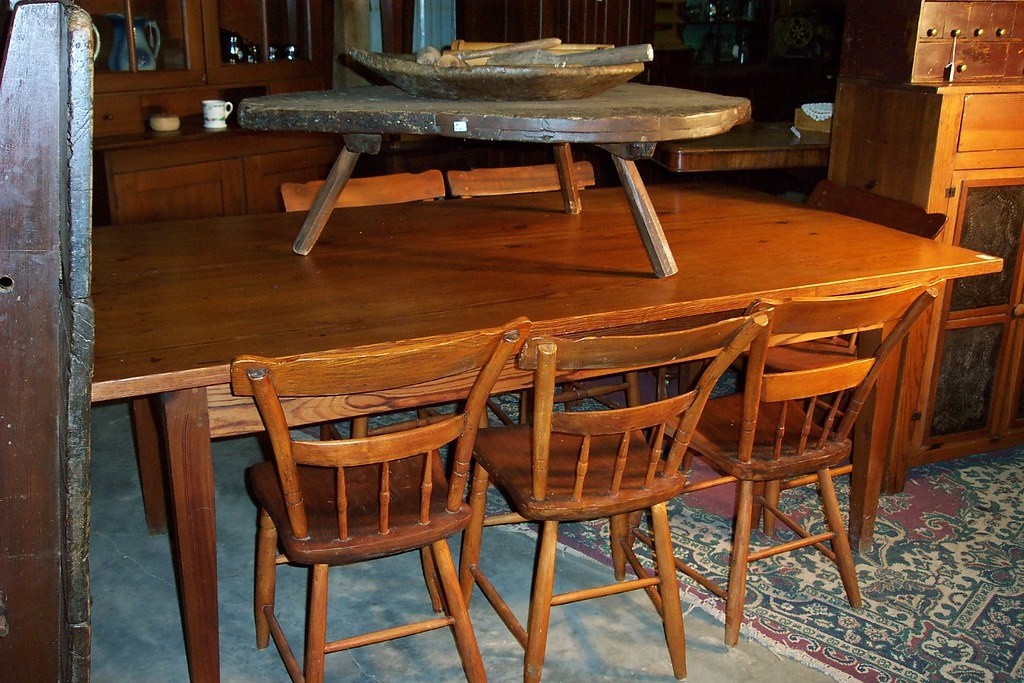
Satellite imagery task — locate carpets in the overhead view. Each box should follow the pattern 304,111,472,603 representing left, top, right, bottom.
301,386,1024,683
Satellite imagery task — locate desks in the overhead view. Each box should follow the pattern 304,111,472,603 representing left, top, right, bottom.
654,121,831,173
241,81,752,279
93,182,1006,683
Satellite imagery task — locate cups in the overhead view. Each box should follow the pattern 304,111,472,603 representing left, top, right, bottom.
201,99,233,129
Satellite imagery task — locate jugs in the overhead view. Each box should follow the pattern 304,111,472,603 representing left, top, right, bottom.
106,14,160,71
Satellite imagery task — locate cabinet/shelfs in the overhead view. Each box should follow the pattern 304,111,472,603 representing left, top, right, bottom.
72,0,345,221
461,0,848,184
826,79,1024,496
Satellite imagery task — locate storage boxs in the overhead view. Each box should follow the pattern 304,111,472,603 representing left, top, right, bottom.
794,108,834,133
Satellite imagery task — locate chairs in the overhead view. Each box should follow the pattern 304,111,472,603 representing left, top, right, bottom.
666,283,940,648
228,318,534,683
465,308,774,683
